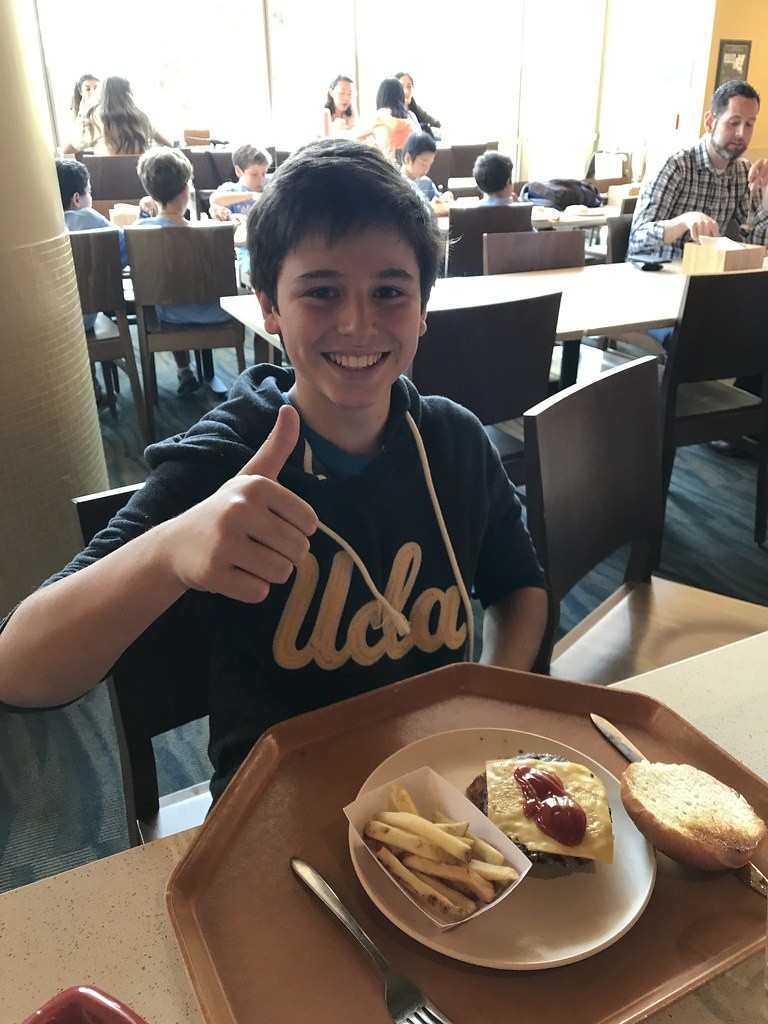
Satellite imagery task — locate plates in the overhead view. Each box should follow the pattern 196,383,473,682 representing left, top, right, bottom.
347,728,656,969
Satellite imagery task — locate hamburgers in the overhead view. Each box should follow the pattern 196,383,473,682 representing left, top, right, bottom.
466,751,767,880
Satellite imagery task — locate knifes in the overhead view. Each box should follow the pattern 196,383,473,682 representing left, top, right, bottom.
589,712,768,899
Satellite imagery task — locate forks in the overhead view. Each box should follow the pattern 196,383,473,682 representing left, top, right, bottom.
290,856,456,1024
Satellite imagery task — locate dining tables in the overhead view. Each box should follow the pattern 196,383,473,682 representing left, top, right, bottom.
1,626,768,1024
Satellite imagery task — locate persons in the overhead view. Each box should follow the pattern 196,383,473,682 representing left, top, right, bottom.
52,70,539,415
0,138,548,827
626,81,768,457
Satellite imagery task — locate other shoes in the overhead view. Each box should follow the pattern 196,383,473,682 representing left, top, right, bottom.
98,391,117,412
708,437,756,459
176,377,201,398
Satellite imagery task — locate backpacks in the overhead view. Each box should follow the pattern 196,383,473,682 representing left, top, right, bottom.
520,179,603,211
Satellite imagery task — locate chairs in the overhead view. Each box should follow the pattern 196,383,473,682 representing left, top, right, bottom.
63,140,767,687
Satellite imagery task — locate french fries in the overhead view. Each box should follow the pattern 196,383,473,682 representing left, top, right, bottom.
365,789,520,921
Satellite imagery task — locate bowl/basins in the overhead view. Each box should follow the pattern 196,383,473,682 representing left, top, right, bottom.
343,767,533,930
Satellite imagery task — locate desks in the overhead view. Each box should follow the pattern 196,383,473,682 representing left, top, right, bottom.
436,201,619,240
218,243,767,403
